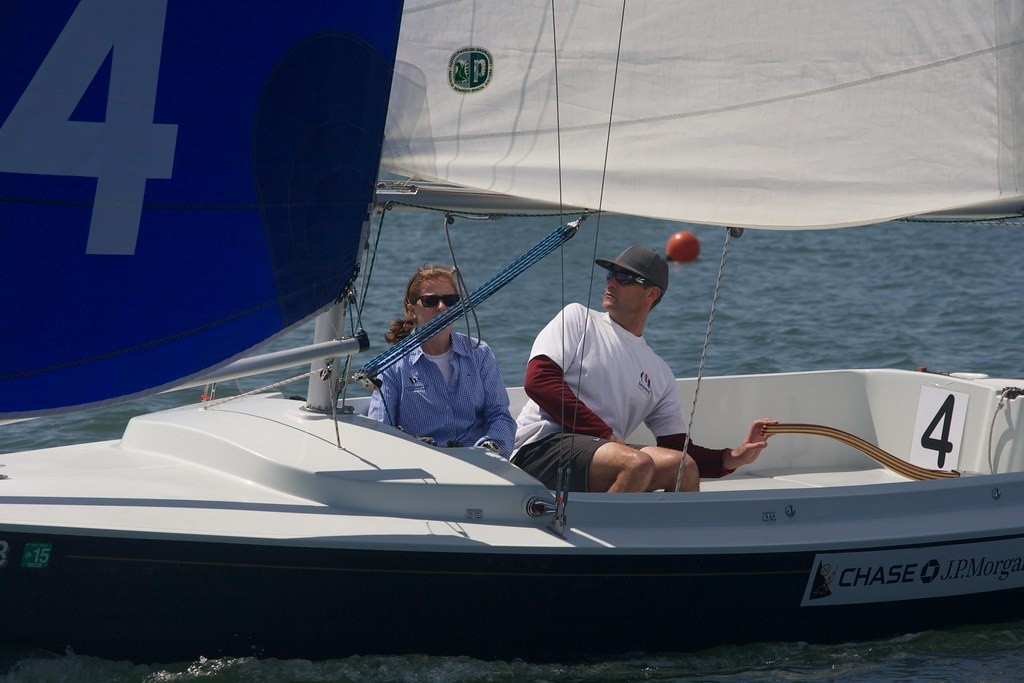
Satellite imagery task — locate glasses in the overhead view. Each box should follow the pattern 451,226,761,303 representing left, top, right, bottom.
607,269,649,288
414,294,460,308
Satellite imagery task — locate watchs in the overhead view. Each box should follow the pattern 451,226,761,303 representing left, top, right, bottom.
481,441,500,454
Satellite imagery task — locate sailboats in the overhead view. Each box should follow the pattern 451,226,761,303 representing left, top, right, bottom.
0,0,1024,621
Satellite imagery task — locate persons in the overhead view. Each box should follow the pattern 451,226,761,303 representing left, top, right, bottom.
367,266,517,460
509,245,778,493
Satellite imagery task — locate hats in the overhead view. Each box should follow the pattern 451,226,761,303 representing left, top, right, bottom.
594,244,669,291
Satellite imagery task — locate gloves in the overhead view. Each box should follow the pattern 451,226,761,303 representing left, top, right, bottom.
480,441,499,455
417,437,437,447
